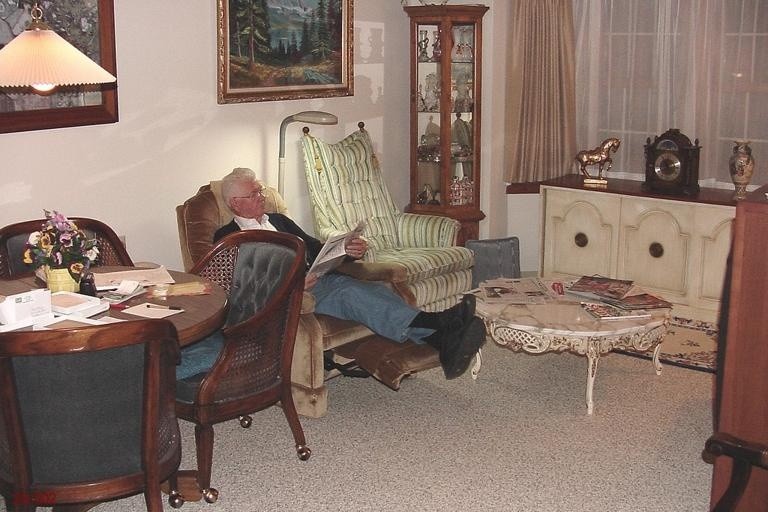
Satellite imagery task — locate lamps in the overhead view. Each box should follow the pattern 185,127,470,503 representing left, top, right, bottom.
0,0,117,96
275,108,339,200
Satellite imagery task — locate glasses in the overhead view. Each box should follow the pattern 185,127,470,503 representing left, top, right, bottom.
233,187,267,201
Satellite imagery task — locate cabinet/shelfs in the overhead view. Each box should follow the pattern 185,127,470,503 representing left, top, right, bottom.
401,3,487,246
706,178,767,511
542,180,621,286
618,191,696,316
695,199,731,312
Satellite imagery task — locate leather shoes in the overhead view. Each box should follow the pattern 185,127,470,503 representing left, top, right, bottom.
432,294,476,349
440,316,486,380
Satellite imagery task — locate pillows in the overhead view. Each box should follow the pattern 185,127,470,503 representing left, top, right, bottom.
459,233,519,281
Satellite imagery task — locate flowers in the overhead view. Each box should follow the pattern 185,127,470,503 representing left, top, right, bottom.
24,207,101,278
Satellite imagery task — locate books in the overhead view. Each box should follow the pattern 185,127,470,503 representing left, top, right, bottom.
1,266,185,334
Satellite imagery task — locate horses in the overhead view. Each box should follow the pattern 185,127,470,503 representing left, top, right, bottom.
575,136,623,180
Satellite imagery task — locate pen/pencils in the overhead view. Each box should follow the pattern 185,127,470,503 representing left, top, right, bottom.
110,304,130,308
147,305,180,310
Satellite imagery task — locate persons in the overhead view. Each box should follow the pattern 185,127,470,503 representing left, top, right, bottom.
565,274,677,322
453,111,474,157
212,165,486,381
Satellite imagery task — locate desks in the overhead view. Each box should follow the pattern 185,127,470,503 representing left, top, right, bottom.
0,262,230,505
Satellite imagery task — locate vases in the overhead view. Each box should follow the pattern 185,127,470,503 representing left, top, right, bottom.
45,264,76,293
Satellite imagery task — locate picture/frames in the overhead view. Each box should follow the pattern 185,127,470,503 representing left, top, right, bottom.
216,0,356,107
0,2,120,133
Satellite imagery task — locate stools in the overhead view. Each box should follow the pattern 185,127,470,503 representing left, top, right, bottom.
698,427,768,512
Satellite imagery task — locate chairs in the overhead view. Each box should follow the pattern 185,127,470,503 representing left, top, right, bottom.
0,318,187,512
162,225,318,501
300,118,472,311
0,212,133,272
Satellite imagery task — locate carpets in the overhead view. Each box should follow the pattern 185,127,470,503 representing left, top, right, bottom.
456,265,722,381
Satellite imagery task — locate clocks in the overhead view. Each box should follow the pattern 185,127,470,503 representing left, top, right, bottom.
639,124,702,198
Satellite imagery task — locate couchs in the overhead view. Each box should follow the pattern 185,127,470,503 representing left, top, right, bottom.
168,178,443,421
